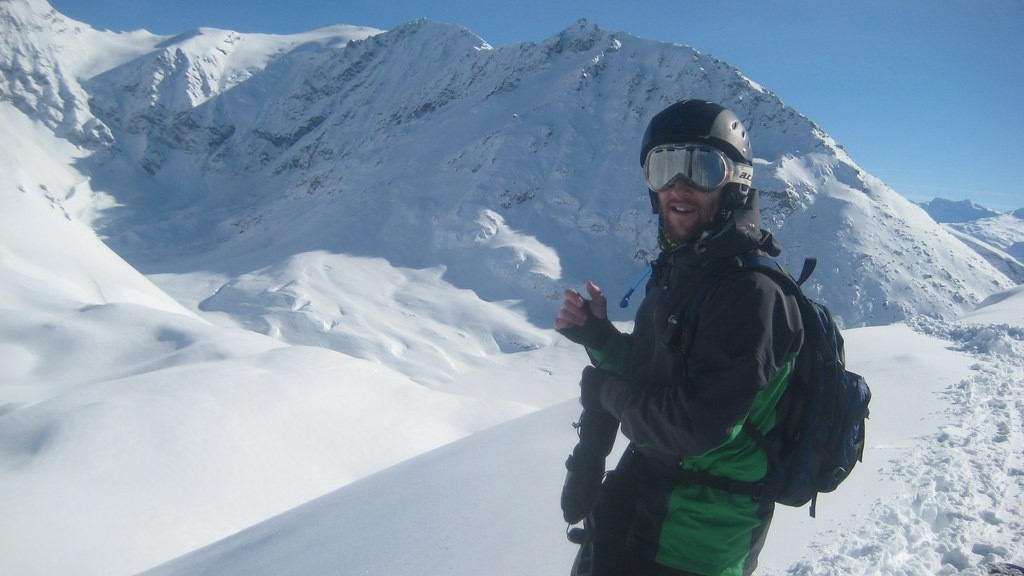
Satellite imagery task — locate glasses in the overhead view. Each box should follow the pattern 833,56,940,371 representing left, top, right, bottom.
644,142,732,194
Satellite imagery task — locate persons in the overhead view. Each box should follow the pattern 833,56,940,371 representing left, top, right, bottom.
555,99,808,576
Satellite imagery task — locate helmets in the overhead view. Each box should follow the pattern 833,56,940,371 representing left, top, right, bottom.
640,98,753,213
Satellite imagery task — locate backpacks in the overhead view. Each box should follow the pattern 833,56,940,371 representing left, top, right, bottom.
663,258,872,520
